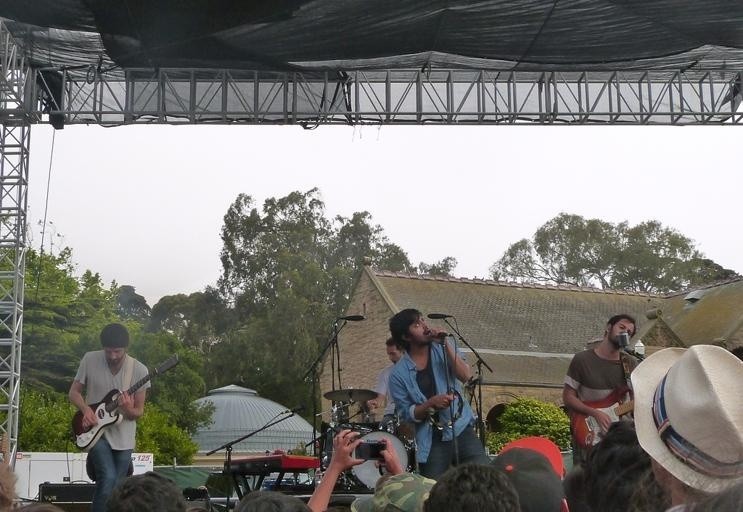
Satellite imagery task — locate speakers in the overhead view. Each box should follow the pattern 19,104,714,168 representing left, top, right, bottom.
39,483,97,501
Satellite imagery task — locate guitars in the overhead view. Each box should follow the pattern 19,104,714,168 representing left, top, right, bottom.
571,385,634,449
73,353,180,451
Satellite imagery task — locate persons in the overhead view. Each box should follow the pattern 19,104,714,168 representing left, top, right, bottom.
228,432,573,512
233,429,571,511
390,309,491,480
563,314,637,465
368,337,407,429
68,323,152,512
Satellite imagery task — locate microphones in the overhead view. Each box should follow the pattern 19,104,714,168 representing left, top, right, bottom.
427,314,455,320
340,315,366,321
284,405,305,413
426,330,450,338
615,332,634,354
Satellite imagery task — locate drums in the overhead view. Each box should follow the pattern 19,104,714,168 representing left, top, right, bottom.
346,429,414,490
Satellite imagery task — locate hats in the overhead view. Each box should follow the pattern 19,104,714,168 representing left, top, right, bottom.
351,472,437,512
492,436,565,511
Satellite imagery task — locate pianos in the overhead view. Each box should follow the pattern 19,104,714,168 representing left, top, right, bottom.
224,455,320,471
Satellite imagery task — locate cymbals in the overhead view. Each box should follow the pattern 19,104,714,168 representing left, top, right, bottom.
323,388,379,403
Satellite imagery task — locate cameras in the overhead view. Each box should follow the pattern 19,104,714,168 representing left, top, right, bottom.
356,441,387,459
181,485,208,503
